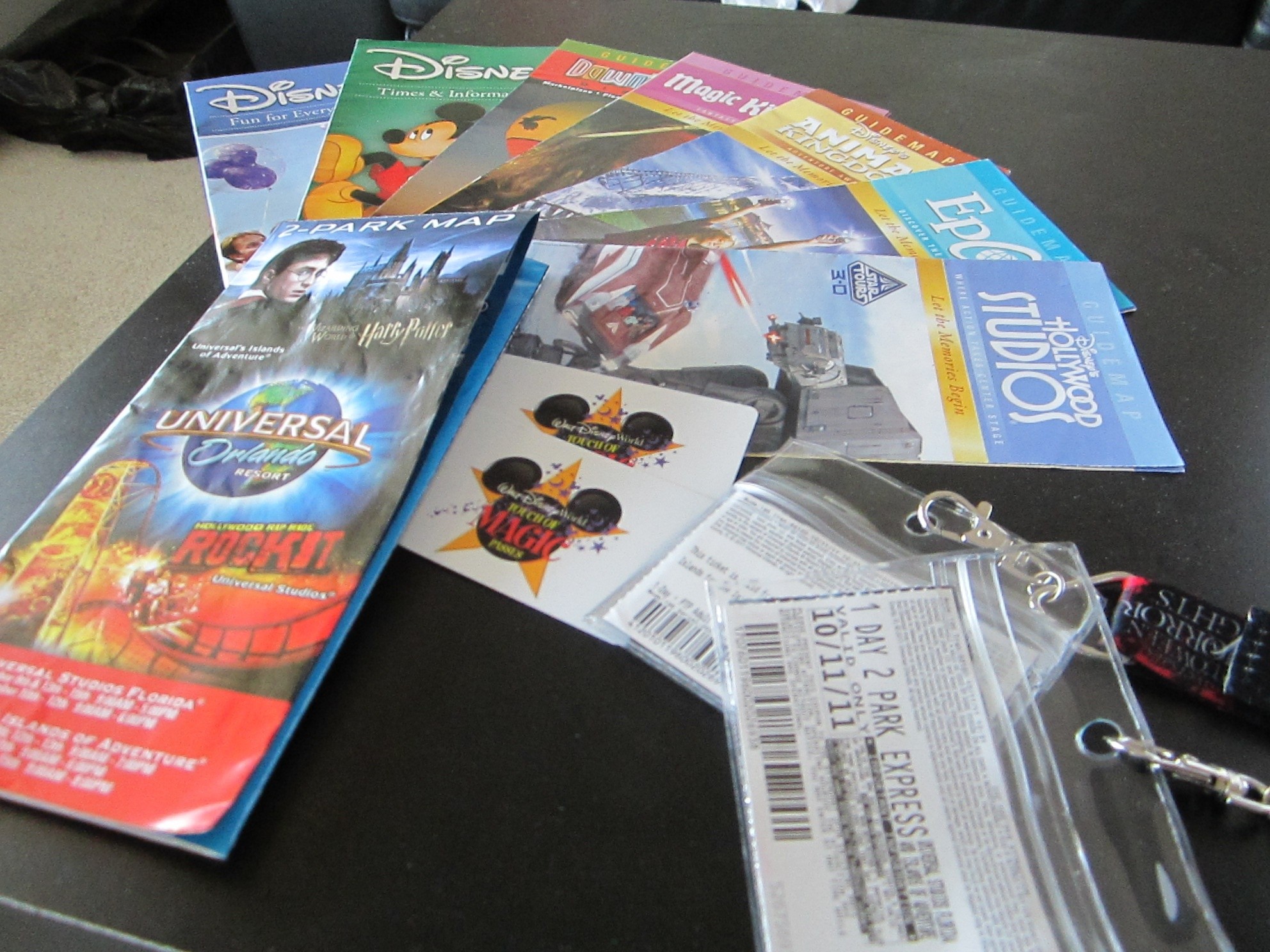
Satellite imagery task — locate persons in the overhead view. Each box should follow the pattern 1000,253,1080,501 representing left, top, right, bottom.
112,240,348,434
222,231,266,275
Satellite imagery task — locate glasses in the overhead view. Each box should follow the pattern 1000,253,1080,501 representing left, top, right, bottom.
282,265,327,282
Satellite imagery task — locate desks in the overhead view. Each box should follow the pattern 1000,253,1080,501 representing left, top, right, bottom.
0,0,1270,952
